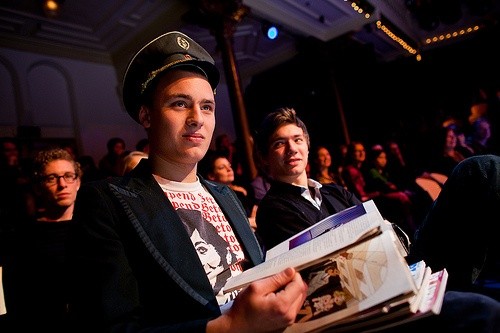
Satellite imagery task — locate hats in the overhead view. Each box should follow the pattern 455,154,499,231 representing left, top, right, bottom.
121,30,221,126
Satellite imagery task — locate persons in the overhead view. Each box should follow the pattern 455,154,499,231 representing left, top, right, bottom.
0,136,44,271
255,106,500,302
309,105,489,205
69,58,500,333
64,137,153,181
196,130,276,238
18,146,84,333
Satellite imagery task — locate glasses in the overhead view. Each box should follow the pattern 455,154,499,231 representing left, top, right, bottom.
39,171,78,184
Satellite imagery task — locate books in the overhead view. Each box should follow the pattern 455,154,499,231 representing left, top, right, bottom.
221,198,448,333
